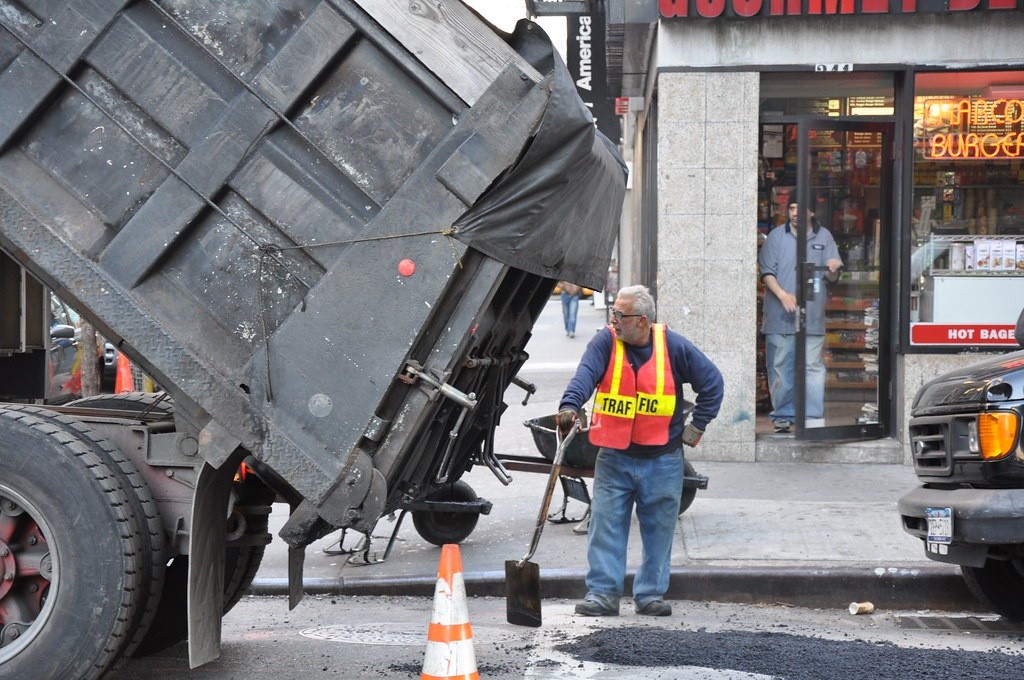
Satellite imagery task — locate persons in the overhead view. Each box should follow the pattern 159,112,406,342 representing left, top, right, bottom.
556,280,581,338
833,195,864,233
760,191,843,429
559,284,724,616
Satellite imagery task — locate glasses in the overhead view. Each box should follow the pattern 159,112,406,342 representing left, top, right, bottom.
608,306,643,320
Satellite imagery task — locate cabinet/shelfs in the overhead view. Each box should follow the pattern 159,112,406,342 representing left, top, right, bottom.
757,186,881,413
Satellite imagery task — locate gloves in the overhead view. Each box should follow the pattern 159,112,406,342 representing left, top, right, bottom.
681,423,704,447
555,409,578,433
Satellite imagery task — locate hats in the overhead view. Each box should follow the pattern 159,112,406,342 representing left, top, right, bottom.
787,187,816,212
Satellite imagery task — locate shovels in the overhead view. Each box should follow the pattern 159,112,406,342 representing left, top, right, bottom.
504,412,582,627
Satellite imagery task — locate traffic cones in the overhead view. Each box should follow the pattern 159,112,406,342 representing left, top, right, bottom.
419,545,481,680
114,348,135,394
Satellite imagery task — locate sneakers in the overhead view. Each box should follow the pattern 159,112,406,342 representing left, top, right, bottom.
575,600,620,616
636,600,671,616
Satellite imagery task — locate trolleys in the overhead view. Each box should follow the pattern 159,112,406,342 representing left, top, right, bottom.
322,479,493,566
471,412,708,534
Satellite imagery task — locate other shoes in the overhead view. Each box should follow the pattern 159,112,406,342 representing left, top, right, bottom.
774,426,791,433
569,330,576,338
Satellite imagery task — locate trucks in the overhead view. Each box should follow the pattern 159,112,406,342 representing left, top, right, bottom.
0,0,628,680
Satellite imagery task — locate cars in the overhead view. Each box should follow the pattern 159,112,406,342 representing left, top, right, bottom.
9,292,106,405
553,280,593,300
898,308,1024,623
105,340,161,392
